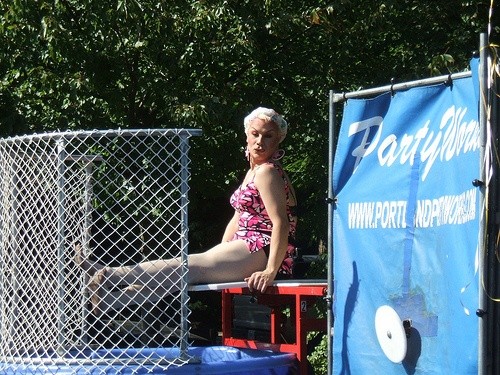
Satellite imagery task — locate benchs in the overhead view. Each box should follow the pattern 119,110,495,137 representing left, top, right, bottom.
221,284,328,375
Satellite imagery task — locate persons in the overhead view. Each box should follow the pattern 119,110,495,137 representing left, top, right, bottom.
75,107,297,316
